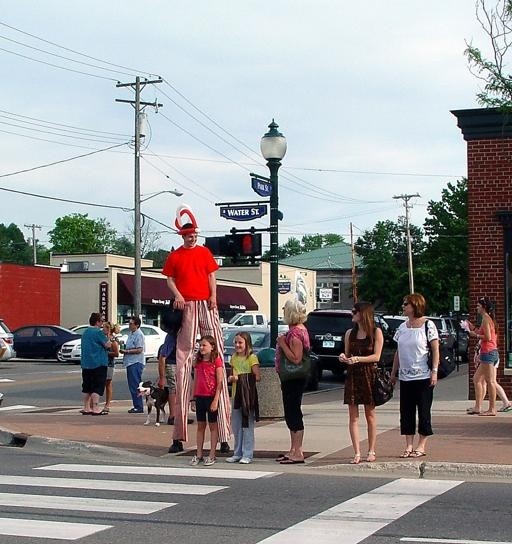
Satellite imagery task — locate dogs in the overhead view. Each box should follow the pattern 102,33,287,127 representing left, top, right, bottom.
135,379,168,426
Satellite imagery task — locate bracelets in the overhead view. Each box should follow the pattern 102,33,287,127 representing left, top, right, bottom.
354,356,360,363
431,368,439,374
476,334,480,338
125,349,129,354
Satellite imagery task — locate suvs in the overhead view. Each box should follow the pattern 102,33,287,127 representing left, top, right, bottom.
305,307,471,390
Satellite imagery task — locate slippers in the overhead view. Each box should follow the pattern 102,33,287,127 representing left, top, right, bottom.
275,453,290,462
78,405,112,416
466,403,512,416
280,456,305,465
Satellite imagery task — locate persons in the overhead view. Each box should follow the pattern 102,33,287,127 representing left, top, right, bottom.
119,315,145,413
339,303,385,462
156,315,192,424
391,293,439,459
80,312,110,415
276,298,310,464
100,322,121,412
467,305,511,412
469,296,499,416
225,331,260,463
162,205,232,455
190,335,224,465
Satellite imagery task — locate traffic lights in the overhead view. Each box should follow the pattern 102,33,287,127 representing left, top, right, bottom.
227,233,263,254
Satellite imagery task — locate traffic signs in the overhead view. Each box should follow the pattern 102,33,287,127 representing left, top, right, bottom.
250,177,273,195
219,203,268,221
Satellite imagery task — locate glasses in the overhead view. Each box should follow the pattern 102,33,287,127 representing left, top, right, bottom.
352,310,360,315
403,302,412,306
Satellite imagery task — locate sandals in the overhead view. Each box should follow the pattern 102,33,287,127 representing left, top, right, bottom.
408,450,427,457
350,454,362,464
202,455,217,466
365,450,377,462
188,454,205,466
399,447,413,459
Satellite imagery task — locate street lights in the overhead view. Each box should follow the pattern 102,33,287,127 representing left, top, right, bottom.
259,117,287,348
134,190,184,316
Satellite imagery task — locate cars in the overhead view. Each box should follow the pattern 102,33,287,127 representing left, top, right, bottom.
1,311,290,395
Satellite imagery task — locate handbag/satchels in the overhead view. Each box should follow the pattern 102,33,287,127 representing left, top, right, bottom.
424,319,456,380
372,367,394,407
278,348,312,382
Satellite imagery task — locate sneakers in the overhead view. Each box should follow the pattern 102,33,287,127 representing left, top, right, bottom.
167,416,194,426
238,456,253,464
127,407,144,413
168,438,184,453
225,456,242,463
220,442,230,453
